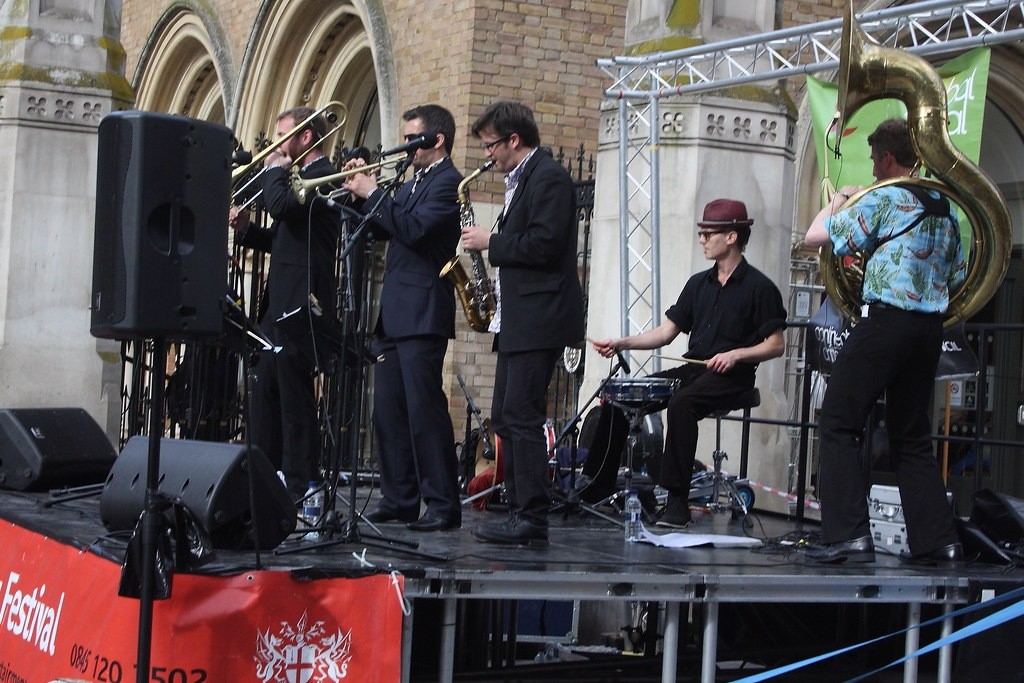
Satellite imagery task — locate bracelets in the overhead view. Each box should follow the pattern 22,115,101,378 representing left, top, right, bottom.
367,189,378,197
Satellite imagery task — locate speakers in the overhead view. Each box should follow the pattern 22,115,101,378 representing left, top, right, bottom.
98,434,297,552
90,110,233,344
968,489,1024,566
0,407,118,491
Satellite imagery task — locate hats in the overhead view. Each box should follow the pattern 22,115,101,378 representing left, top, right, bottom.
698,198,754,230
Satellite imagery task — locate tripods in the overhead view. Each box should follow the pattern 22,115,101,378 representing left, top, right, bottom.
575,408,654,526
273,150,448,560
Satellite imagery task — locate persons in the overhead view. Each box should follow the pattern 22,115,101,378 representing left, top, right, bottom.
461,101,586,546
229,107,343,508
341,104,465,531
804,117,966,569
578,198,787,528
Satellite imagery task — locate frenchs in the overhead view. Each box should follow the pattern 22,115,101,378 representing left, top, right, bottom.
817,0,1014,336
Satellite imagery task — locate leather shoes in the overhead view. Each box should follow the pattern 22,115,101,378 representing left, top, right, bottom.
357,506,418,523
804,534,875,563
405,509,462,530
900,543,964,571
470,512,550,547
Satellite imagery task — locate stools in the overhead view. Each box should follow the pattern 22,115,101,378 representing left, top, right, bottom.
659,387,760,528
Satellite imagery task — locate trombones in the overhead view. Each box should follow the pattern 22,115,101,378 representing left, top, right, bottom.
229,101,349,223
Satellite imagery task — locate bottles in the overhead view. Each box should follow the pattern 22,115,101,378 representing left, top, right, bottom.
624,491,642,542
303,481,322,540
641,467,648,477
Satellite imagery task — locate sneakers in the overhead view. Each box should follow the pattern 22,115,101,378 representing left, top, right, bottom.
655,501,691,527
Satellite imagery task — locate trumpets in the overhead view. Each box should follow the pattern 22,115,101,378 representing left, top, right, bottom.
291,153,408,205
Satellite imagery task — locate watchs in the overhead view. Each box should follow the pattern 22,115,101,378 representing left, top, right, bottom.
839,190,849,200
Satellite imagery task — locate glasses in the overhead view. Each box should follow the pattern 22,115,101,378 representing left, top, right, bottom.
480,135,510,153
403,132,423,140
698,229,735,243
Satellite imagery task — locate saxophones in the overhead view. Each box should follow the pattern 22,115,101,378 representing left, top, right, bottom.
440,159,497,334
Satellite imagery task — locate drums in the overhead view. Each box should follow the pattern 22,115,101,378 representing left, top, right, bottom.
475,417,557,507
601,377,678,415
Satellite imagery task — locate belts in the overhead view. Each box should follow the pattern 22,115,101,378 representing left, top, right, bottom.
869,300,894,309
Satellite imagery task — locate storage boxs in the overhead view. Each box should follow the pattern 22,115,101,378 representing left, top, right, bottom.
867,485,953,558
490,597,580,645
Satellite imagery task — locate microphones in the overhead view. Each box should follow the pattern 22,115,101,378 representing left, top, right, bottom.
614,347,631,374
232,150,252,165
320,197,364,222
380,131,437,156
